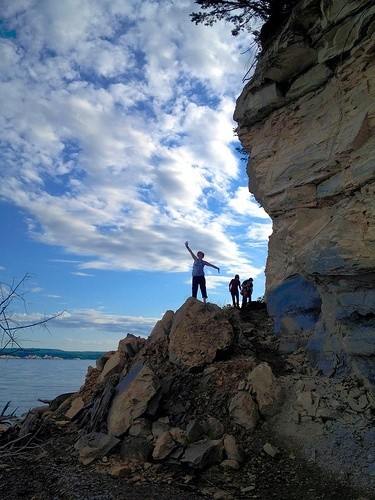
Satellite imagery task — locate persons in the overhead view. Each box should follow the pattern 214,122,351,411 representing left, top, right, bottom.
229,274,242,308
241,278,254,306
185,240,220,304
